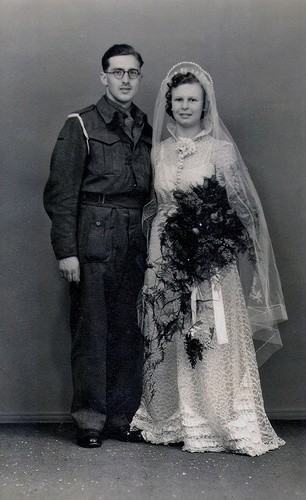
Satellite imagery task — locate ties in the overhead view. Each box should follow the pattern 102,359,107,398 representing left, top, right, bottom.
124,110,135,132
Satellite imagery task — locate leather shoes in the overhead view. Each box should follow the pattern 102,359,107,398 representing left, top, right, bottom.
107,424,141,442
78,426,102,447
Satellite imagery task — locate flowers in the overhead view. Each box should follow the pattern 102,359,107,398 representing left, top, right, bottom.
137,175,255,408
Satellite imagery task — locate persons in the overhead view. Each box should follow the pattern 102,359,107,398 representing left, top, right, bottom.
42,44,155,447
130,62,288,457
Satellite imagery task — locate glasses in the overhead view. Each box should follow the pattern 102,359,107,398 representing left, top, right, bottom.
106,69,141,80
